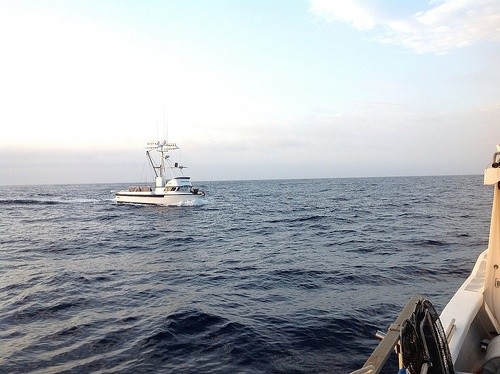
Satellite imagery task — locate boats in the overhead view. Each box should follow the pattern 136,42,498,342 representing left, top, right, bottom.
353,143,500,374
111,138,204,205
